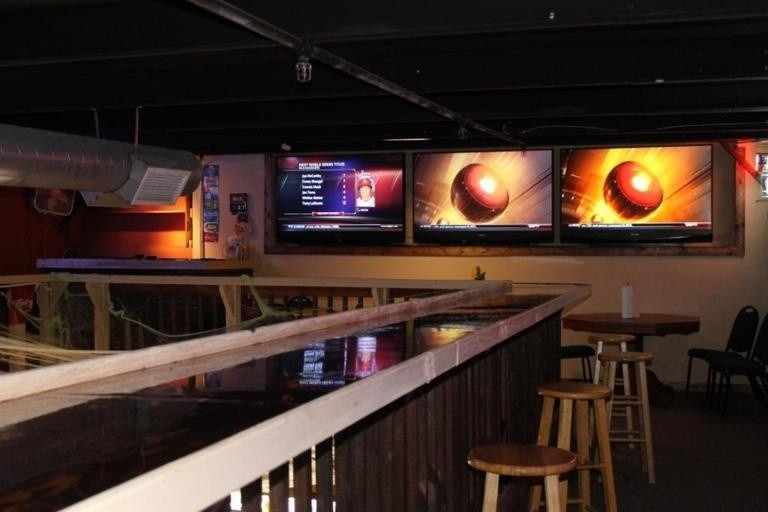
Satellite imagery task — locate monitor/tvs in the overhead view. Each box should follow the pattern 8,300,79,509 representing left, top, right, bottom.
410,149,555,245
411,311,509,360
274,318,408,396
558,144,715,244
274,151,408,247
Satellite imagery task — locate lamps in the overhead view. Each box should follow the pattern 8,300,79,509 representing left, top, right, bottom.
295,56,313,82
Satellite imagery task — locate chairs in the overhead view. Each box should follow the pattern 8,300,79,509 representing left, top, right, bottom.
687,304,758,397
559,346,596,383
706,314,768,397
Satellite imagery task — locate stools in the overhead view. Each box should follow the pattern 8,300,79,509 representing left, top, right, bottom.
588,334,635,449
593,352,656,485
466,443,578,512
527,379,617,511
